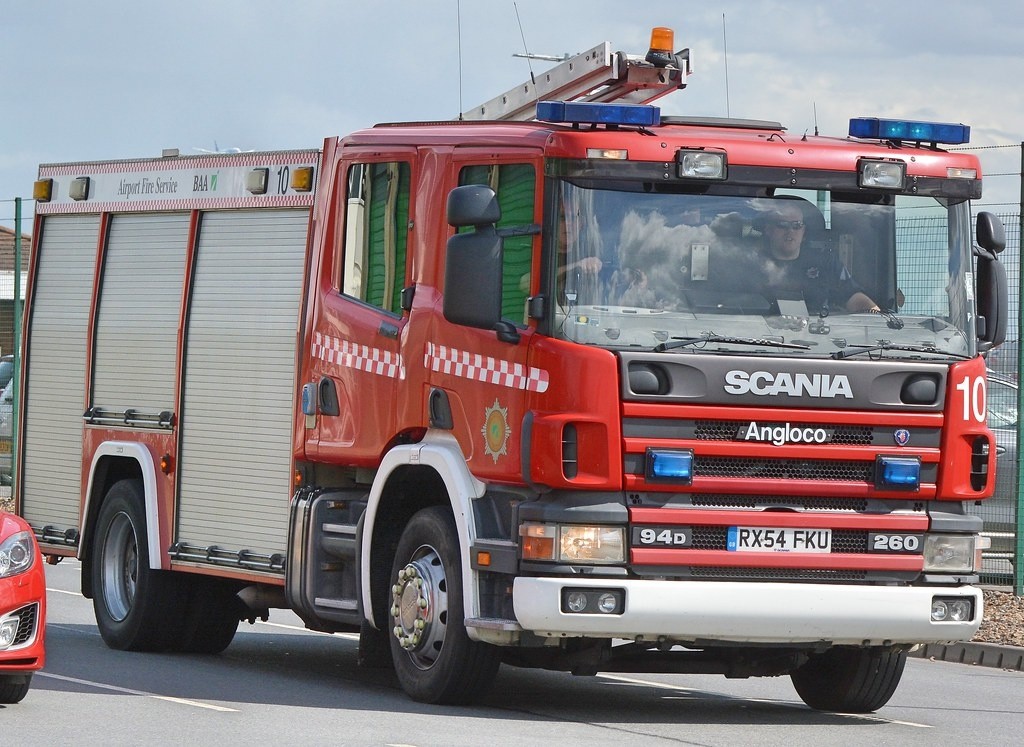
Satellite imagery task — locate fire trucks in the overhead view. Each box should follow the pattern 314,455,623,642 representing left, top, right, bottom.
15,25,1005,718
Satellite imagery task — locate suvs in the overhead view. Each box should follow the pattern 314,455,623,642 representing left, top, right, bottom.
0,356,13,485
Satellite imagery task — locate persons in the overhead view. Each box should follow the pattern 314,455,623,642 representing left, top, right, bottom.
519,200,647,310
730,204,883,315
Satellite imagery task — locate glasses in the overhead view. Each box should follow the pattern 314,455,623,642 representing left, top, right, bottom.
560,214,578,222
768,220,804,230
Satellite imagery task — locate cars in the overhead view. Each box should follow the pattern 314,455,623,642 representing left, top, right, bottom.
0,507,47,707
979,368,1018,564
